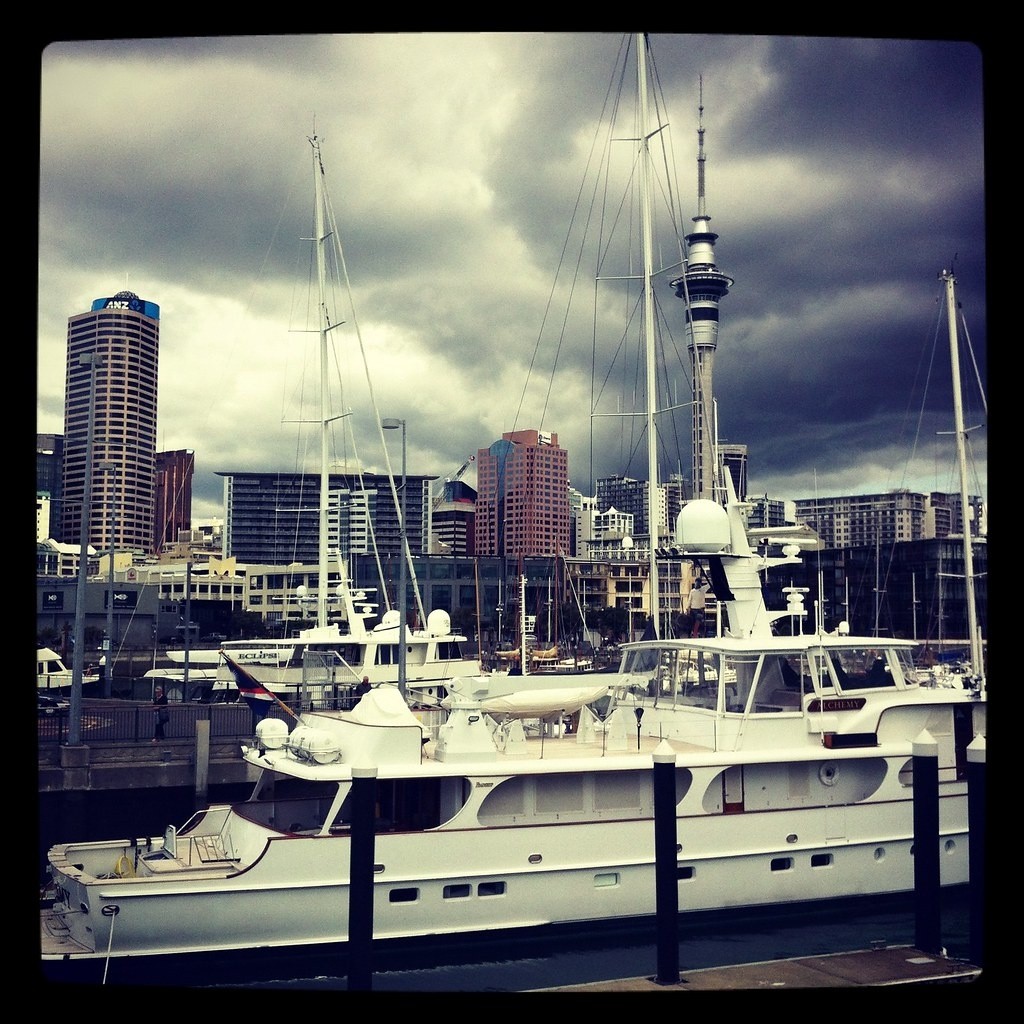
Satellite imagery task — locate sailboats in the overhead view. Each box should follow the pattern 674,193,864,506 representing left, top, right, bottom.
13,35,990,993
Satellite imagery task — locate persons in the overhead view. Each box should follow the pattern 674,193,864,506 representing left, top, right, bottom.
356,676,373,705
152,686,170,741
826,659,852,689
686,577,713,639
871,652,887,687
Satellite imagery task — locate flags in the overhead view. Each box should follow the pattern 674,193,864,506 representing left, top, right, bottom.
221,652,280,719
